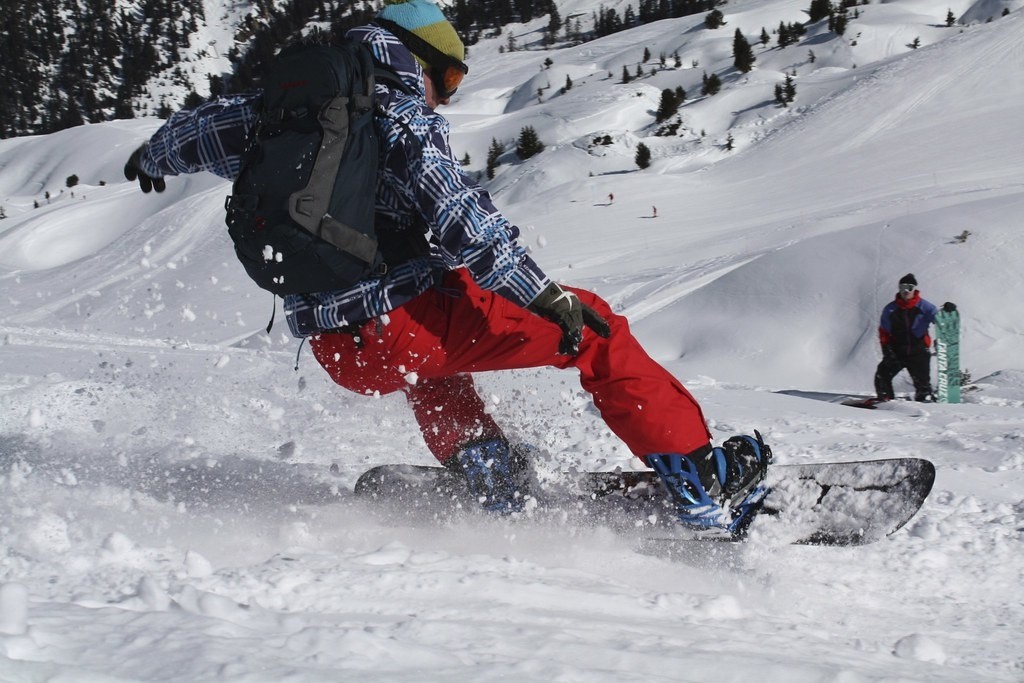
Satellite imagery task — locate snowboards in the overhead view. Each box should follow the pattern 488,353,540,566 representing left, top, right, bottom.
354,456,936,547
934,309,961,405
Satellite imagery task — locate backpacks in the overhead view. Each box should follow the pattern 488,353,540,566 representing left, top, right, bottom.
225,42,428,335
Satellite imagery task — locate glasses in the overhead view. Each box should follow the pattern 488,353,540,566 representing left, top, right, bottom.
373,17,468,100
898,284,915,292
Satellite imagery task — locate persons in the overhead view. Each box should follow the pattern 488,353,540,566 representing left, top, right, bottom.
124,1,772,542
873,273,938,403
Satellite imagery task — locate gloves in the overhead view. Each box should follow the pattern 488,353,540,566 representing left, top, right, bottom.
525,283,610,355
124,145,165,193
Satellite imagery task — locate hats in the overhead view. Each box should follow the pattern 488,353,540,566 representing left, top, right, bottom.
899,273,918,286
372,0,464,70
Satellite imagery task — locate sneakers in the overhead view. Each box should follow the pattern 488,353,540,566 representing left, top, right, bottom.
441,434,541,516
644,430,772,535
